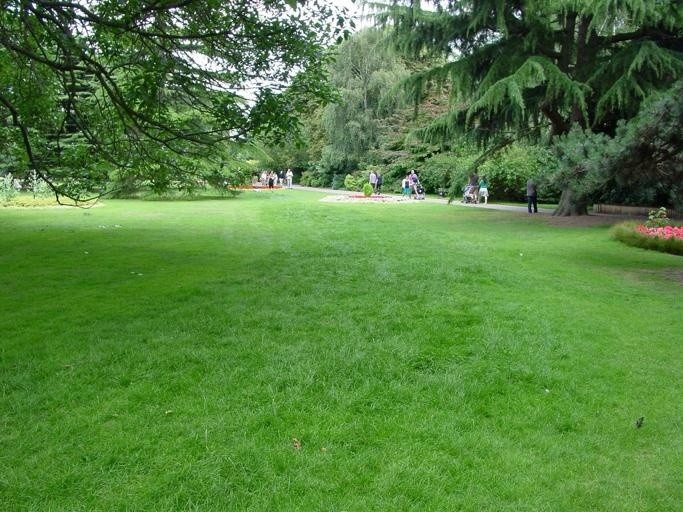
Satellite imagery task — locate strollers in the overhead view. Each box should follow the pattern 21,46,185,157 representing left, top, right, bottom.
412,183,425,200
461,185,479,204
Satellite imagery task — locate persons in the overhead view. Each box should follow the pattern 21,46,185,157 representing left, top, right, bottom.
468,173,479,204
369,171,376,189
374,173,382,195
472,178,489,204
527,179,538,213
409,170,423,197
261,169,293,189
402,175,413,198
